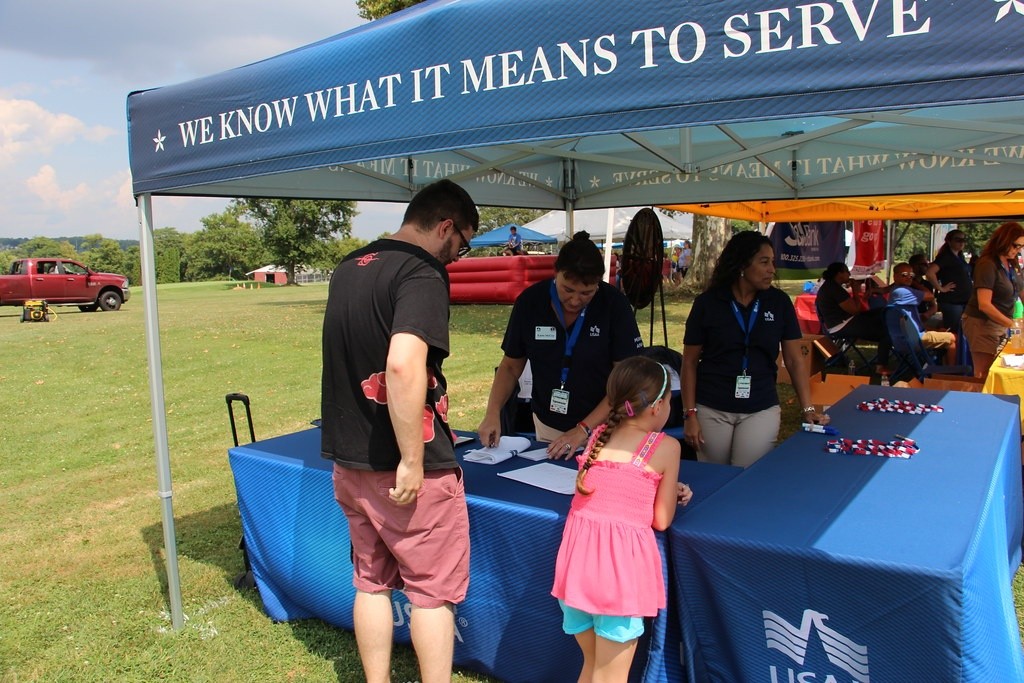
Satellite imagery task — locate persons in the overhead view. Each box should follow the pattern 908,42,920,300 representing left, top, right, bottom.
551,355,692,683
480,230,645,460
817,221,1024,389
318,181,479,683
681,231,831,468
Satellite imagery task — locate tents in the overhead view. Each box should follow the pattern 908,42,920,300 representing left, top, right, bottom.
655,189,1024,230
123,0,1023,634
526,206,697,249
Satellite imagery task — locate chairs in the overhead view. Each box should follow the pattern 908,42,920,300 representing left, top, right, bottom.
48,266,54,272
815,298,972,379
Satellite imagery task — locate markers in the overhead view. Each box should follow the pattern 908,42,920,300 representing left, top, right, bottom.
803,427,839,435
801,423,837,430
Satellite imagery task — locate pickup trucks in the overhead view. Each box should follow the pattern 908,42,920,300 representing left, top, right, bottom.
0,257,131,315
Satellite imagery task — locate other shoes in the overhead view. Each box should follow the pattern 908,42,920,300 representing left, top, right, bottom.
875,364,898,375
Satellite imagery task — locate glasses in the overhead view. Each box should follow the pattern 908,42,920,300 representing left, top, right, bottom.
919,263,927,267
1012,243,1024,249
439,216,472,257
954,237,966,243
896,272,915,279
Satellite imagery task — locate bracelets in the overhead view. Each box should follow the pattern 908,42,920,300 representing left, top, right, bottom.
681,408,698,420
576,421,592,435
800,406,816,414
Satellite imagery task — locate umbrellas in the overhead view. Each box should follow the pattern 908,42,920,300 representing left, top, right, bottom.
469,220,558,251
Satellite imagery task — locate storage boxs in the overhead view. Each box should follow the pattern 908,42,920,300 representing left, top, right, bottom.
775,325,987,414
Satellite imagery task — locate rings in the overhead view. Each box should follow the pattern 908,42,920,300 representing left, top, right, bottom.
566,444,571,450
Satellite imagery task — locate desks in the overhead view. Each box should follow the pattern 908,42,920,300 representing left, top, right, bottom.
227,385,1024,683
982,342,1024,436
794,285,890,334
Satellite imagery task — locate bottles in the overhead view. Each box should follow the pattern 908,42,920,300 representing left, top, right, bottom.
1010,318,1024,349
1013,297,1023,319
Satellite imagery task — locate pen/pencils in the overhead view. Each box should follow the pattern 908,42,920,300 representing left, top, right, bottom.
893,433,917,444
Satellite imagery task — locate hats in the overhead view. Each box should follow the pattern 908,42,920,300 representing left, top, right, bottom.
673,244,680,247
